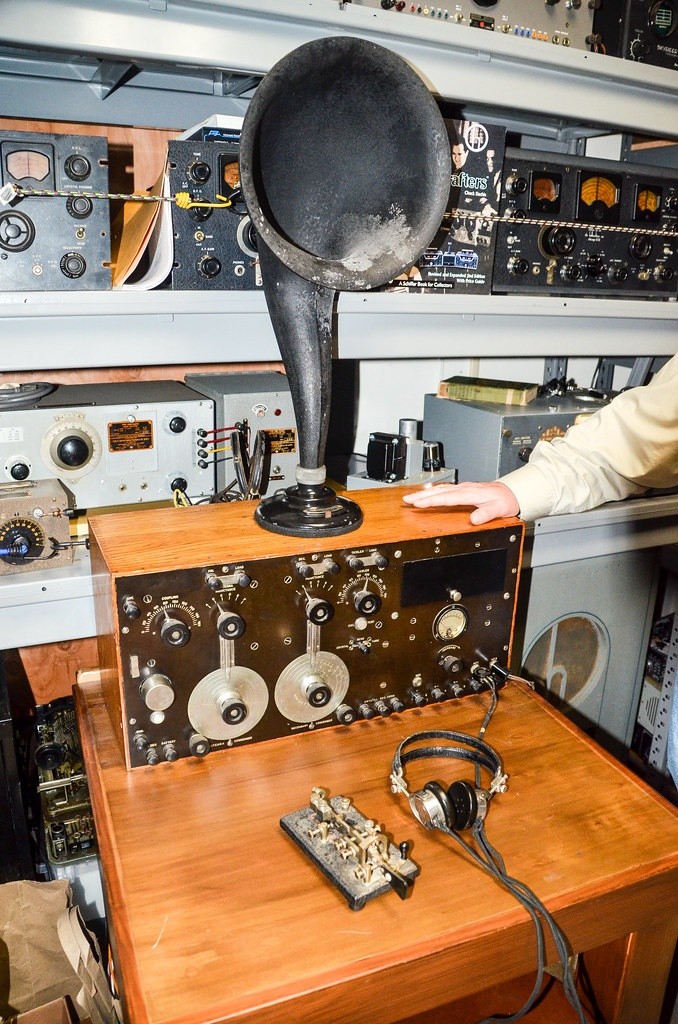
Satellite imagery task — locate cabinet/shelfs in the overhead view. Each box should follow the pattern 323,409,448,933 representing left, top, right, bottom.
0,0,678,1024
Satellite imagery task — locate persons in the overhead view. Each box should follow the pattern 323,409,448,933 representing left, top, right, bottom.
400,353,678,526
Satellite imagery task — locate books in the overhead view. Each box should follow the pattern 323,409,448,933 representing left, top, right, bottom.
437,376,539,406
107,165,176,293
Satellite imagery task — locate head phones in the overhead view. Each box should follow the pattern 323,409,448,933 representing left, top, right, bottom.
389,730,508,833
